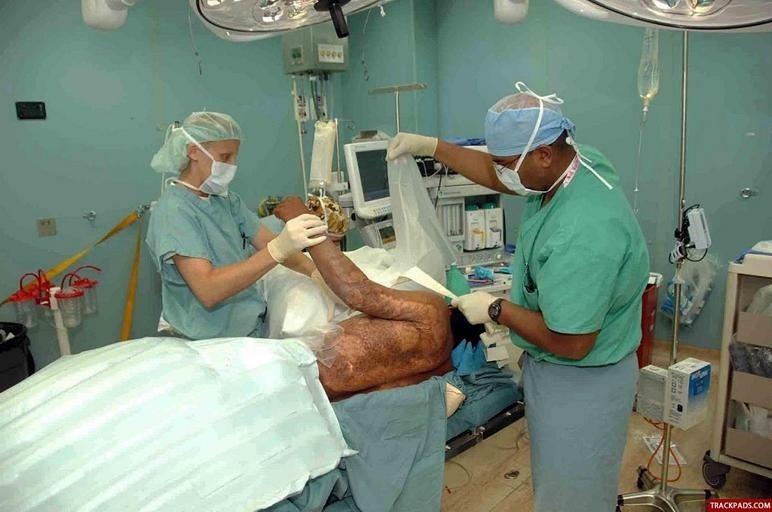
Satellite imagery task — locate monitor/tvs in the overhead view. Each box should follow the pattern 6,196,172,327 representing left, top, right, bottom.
344,141,392,218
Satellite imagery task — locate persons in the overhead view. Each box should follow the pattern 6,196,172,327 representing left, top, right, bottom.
383,82,651,512
142,109,329,340
273,196,485,400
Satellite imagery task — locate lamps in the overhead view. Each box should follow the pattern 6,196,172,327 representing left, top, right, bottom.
189,1,392,42
554,1,771,34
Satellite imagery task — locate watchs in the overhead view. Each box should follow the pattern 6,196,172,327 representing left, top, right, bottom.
488,297,505,324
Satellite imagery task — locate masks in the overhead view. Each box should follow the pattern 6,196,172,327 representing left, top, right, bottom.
198,159,238,199
493,163,548,199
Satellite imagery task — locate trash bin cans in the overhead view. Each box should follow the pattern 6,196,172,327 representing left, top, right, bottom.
637,272,664,367
0,321,26,393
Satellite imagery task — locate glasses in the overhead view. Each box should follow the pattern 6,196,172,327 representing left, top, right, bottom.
492,153,522,175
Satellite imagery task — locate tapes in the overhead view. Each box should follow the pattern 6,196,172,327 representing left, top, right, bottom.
257,196,280,216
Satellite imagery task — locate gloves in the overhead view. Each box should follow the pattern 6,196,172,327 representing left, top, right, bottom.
309,268,350,322
450,289,504,325
266,214,329,265
384,131,439,161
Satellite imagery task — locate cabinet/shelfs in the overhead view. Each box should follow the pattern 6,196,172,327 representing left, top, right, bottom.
701,246,771,489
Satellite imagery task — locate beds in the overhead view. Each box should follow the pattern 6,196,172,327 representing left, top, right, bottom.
1,330,528,510
